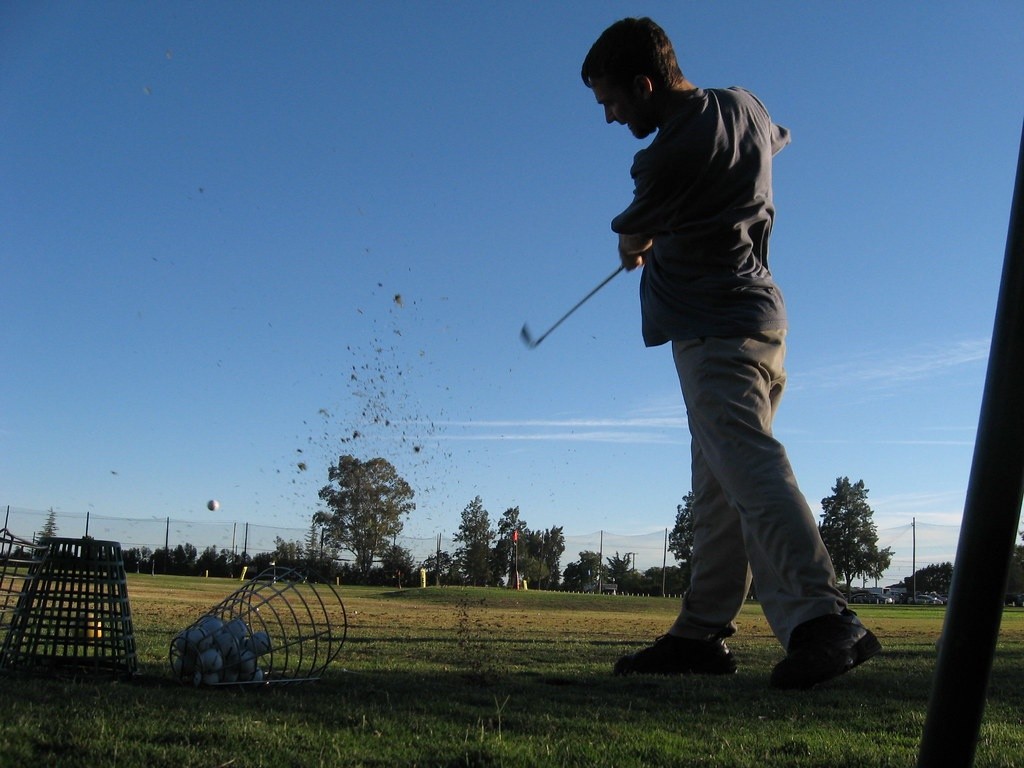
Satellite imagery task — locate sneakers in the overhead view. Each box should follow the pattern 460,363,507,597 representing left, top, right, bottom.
770,610,883,690
614,635,736,677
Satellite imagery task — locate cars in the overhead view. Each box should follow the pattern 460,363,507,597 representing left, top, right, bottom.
885,591,906,604
907,594,947,606
850,592,893,604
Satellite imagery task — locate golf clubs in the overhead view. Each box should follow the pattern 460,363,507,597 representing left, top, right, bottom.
519,263,626,351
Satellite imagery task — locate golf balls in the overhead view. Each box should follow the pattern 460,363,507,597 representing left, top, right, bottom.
172,614,273,686
206,499,220,511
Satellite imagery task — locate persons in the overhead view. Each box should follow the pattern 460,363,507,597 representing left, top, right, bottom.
579,16,882,689
